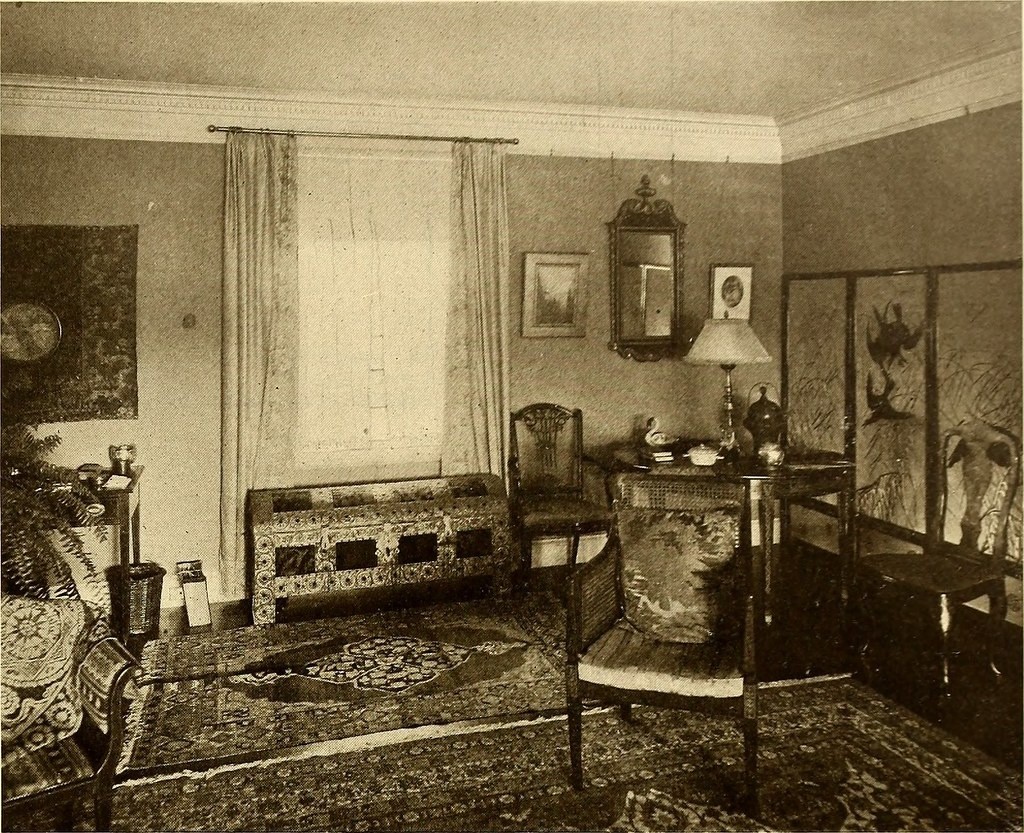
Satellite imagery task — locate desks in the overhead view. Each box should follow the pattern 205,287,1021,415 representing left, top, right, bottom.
584,435,857,632
39,465,145,650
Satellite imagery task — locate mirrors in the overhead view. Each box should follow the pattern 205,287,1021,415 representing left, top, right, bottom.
602,175,688,363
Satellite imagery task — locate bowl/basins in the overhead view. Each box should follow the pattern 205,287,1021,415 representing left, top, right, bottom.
683,444,724,467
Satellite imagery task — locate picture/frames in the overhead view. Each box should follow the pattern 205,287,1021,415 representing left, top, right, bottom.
710,265,753,324
520,251,588,337
780,270,854,560
928,258,1024,581
852,266,931,544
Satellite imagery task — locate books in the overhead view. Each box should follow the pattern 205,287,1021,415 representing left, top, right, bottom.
639,446,674,461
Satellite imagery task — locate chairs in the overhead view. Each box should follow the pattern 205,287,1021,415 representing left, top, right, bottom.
510,401,613,593
569,474,754,797
0,592,140,833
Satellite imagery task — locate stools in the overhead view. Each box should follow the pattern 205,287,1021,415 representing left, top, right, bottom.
863,553,1008,696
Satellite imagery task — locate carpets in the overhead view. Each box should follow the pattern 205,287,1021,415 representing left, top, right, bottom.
114,595,851,786
98,673,1024,833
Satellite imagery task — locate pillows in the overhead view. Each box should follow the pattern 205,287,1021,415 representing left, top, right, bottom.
614,498,746,647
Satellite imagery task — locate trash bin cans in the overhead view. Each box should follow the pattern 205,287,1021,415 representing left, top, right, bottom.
103,564,168,635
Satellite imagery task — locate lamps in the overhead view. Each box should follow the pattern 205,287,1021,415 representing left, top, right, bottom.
684,319,772,454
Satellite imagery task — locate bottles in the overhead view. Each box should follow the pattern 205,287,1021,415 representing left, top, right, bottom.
759,441,782,470
